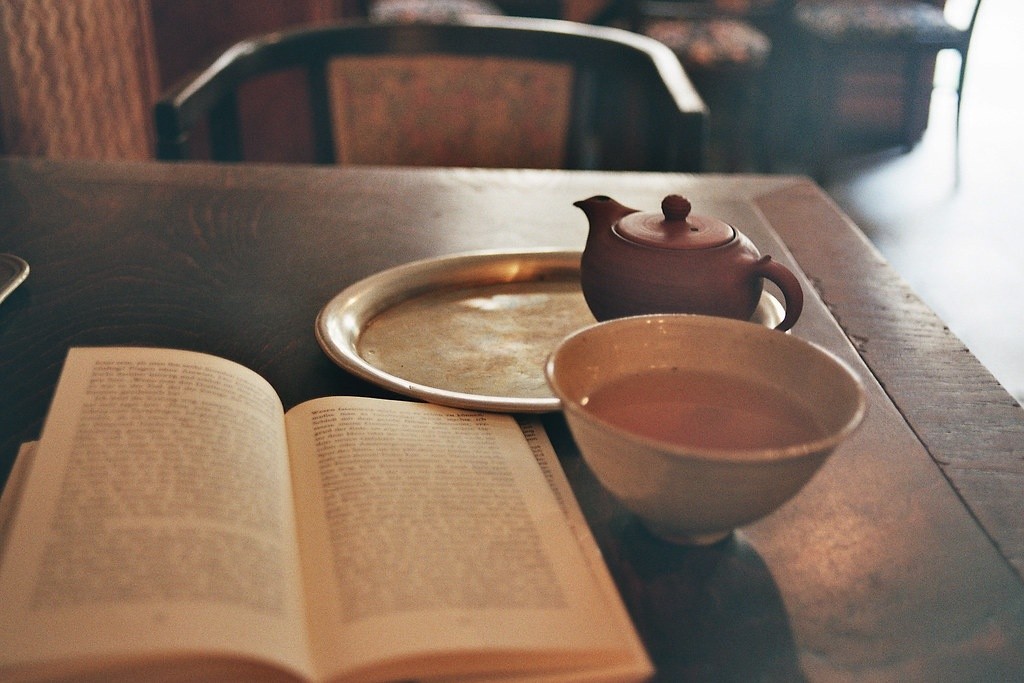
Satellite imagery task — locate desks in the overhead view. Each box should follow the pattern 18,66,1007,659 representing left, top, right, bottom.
0,171,1023,682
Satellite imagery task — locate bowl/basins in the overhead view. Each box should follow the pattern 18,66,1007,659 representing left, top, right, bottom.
544,314,867,547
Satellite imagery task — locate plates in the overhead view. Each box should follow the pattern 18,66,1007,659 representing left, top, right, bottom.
314,245,791,417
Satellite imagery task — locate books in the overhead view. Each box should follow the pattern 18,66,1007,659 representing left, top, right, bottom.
0,347,656,683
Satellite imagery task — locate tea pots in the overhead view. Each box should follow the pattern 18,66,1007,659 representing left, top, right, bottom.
573,192,802,348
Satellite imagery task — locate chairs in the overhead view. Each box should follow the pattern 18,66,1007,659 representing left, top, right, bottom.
155,12,709,179
